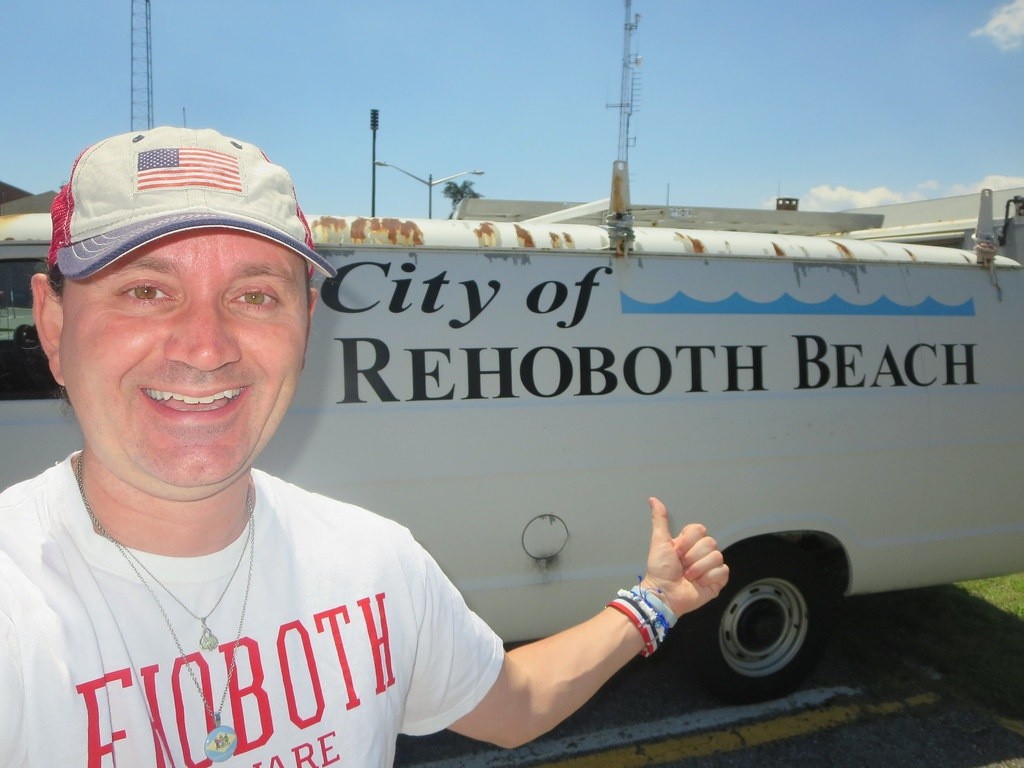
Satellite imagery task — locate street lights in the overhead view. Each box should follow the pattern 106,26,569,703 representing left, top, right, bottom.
372,160,484,221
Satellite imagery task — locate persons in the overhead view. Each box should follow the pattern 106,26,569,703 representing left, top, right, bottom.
0,124,732,768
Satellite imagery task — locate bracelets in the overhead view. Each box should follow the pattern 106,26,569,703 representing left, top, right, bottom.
606,575,678,658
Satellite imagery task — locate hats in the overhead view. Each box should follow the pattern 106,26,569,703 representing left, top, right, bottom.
49,127,339,280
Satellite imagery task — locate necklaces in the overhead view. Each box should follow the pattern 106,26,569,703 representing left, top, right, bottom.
75,447,258,763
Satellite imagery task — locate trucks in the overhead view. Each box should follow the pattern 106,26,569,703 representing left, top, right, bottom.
0,206,1024,697
